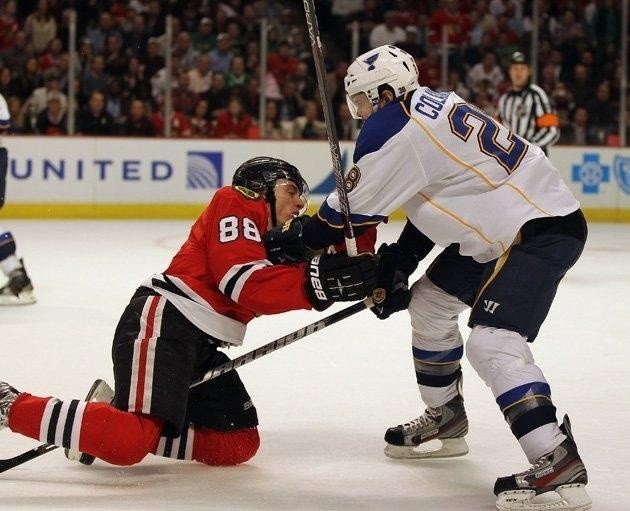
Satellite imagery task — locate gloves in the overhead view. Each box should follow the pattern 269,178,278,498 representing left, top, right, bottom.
261,213,364,265
303,243,419,320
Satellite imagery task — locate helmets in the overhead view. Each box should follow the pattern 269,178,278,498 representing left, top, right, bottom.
342,45,420,121
233,156,311,216
509,50,527,63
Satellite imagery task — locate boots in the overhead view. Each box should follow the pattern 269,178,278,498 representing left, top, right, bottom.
0,257,33,297
0,381,21,431
65,379,118,464
385,371,468,446
494,414,588,497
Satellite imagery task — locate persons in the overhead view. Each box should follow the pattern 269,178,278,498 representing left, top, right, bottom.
0,97,37,298
495,51,560,154
0,151,396,469
1,1,352,140
330,1,622,144
255,40,593,499
